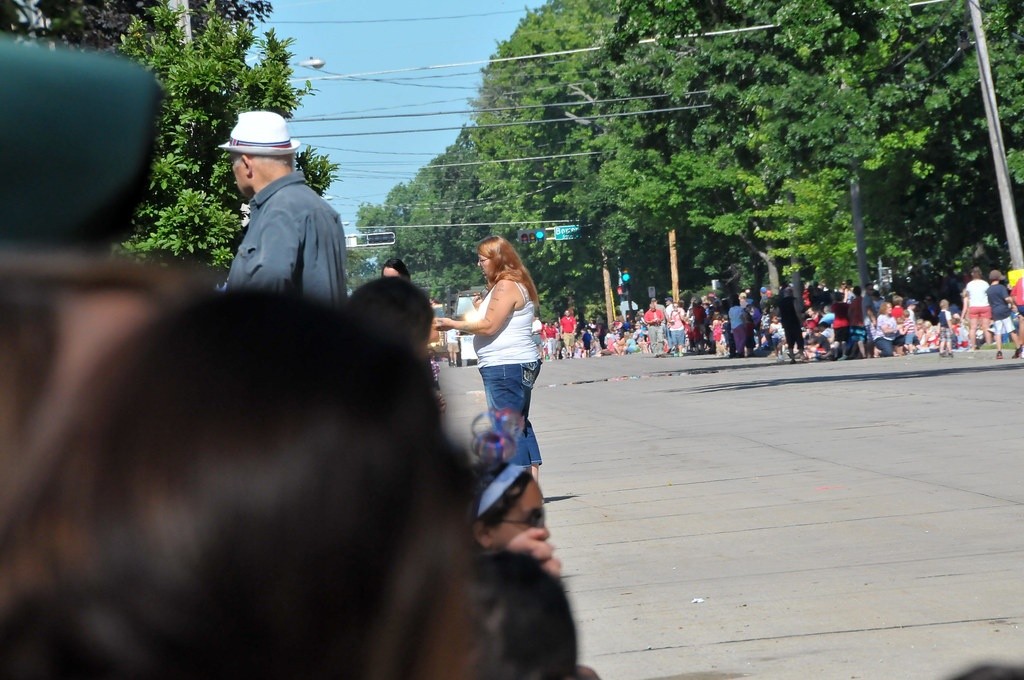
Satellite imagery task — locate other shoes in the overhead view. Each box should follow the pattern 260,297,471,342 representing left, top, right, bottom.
939,352,953,358
997,351,1003,359
1012,348,1024,359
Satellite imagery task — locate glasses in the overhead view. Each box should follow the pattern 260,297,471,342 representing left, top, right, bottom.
499,509,544,529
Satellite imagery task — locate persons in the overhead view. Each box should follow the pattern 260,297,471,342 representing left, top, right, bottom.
0,248,603,680
445,329,460,365
218,110,349,313
532,266,1024,364
382,235,543,487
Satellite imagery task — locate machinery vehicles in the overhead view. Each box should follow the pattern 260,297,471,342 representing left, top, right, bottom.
442,284,481,367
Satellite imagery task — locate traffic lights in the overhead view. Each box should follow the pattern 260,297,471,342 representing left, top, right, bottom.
518,228,546,244
621,272,632,284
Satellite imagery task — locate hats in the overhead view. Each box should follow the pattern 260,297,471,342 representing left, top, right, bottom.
760,287,767,293
218,111,301,156
665,297,673,302
989,270,1004,280
